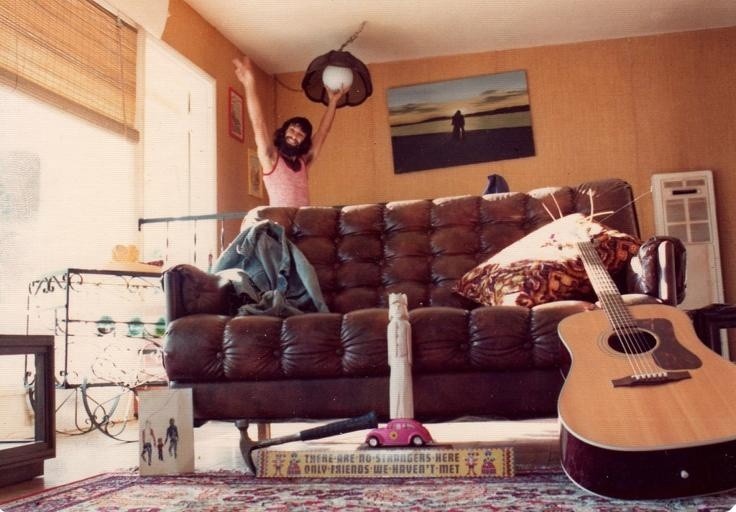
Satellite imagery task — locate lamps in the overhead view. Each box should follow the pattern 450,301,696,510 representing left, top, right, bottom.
301,17,375,112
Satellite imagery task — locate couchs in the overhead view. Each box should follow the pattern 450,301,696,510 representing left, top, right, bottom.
160,175,686,428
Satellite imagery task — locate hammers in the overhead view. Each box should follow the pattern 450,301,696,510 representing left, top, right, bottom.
235,412,378,475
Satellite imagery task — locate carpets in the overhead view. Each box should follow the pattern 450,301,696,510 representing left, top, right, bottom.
1,467,736,512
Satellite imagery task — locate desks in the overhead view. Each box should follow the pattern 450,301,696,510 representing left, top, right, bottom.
682,304,736,359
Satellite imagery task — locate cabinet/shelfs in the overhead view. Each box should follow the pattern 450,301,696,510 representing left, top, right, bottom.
0,329,58,488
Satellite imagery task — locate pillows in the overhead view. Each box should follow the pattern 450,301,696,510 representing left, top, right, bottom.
450,212,641,309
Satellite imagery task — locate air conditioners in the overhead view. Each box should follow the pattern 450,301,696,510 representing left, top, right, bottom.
652,167,731,362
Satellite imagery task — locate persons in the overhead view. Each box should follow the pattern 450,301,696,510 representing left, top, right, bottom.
142,419,154,465
452,113,458,138
157,437,165,460
387,292,416,419
166,417,179,458
232,55,350,207
457,110,464,136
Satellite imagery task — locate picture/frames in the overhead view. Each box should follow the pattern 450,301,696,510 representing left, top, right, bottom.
227,84,269,200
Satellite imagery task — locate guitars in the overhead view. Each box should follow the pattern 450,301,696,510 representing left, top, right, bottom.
556,213,736,501
